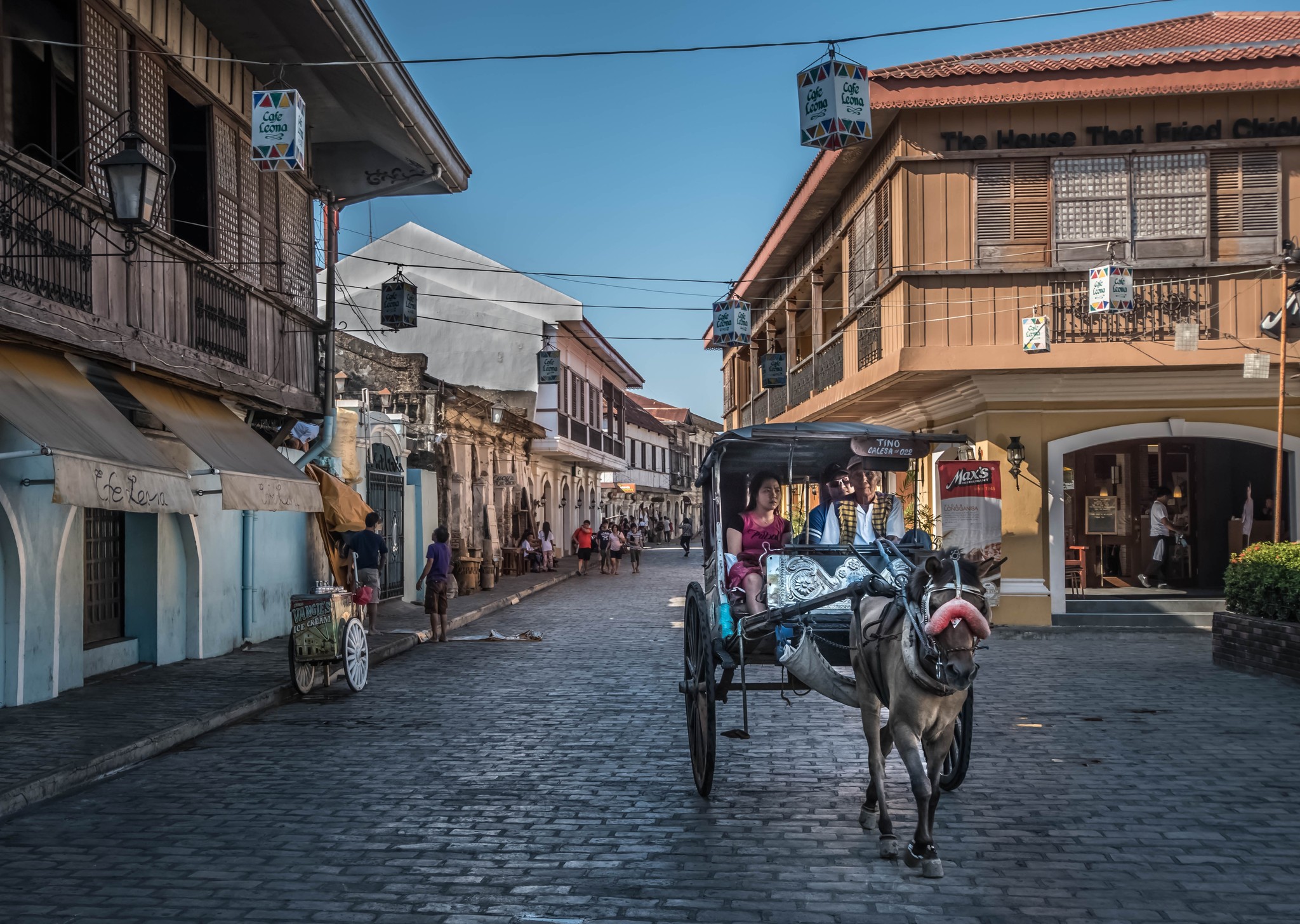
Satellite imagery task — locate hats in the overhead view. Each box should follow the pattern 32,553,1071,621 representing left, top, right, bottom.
846,454,864,470
825,463,850,482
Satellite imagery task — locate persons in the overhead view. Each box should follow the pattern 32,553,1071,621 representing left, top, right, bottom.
521,512,707,576
820,455,905,545
801,462,855,545
347,512,388,636
1262,494,1284,521
1138,486,1184,588
416,526,451,643
726,471,795,618
1241,481,1254,549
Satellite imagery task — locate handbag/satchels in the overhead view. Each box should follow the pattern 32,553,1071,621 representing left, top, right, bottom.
679,534,683,540
620,543,627,554
594,554,602,561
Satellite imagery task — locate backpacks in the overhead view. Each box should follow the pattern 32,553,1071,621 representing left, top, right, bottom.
658,521,664,530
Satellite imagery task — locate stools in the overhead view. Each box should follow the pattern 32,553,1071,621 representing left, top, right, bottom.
1173,545,1192,580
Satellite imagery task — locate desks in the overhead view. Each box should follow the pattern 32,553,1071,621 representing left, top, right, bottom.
502,548,525,578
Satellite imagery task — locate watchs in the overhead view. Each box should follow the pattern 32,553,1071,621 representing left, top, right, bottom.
418,579,423,582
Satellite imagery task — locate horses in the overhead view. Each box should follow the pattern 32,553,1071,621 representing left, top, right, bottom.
848,550,994,879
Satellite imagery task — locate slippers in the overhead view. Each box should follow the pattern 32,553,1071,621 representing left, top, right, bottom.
368,631,383,636
439,637,448,642
425,637,439,643
632,571,636,573
635,567,639,572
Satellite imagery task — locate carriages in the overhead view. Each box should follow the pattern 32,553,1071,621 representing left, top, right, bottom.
674,419,1000,881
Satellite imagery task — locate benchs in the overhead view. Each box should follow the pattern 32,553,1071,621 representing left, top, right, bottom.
704,551,748,619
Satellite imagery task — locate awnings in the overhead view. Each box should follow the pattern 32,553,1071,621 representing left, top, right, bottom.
0,342,197,515
104,365,325,512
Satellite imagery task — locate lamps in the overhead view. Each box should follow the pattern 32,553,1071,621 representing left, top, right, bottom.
956,442,976,461
0,109,176,257
659,476,682,487
538,492,547,509
1111,465,1122,485
1173,472,1182,498
575,496,582,509
792,485,800,505
910,459,923,497
1100,485,1108,496
597,499,604,511
441,391,512,432
589,498,596,510
1006,435,1026,492
558,495,567,509
810,484,819,495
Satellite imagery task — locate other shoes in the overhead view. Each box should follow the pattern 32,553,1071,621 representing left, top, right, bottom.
611,568,615,573
576,571,582,576
553,560,558,563
643,542,645,546
600,571,606,574
550,569,557,572
685,551,690,555
584,571,589,574
615,572,619,574
684,554,688,557
532,569,540,573
606,571,610,574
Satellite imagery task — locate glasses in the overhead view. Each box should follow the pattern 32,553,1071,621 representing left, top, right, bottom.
826,476,850,488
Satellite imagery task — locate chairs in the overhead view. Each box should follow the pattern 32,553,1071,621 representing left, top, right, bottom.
513,537,531,572
1065,567,1086,600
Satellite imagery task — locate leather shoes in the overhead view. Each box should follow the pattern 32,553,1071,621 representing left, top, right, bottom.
1158,583,1177,589
1138,573,1151,588
